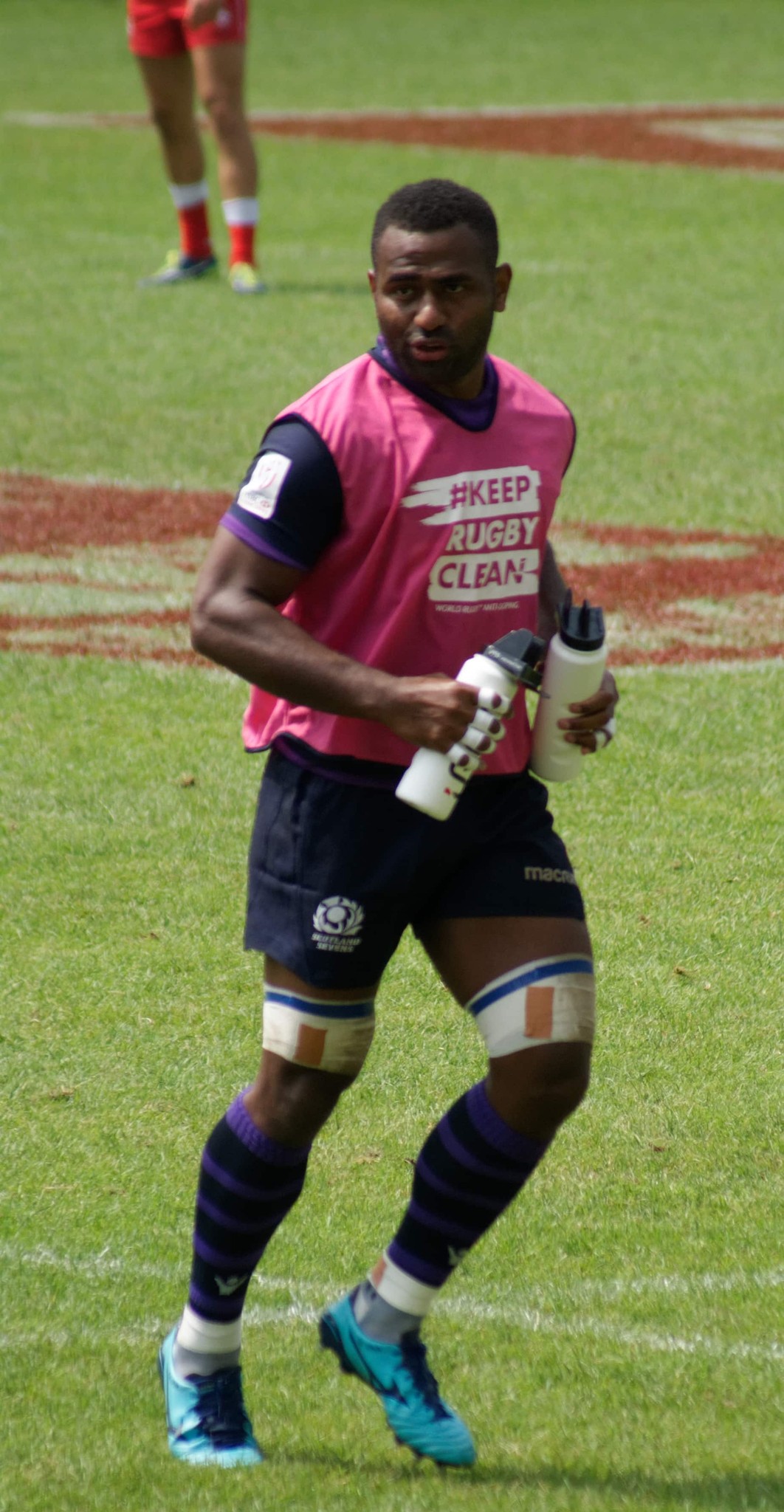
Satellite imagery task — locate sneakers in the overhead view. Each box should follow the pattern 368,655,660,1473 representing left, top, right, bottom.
227,259,266,294
156,1323,261,1471
317,1284,476,1467
146,248,217,284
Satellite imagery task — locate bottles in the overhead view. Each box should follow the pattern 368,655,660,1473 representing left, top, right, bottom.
397,630,544,821
531,590,610,783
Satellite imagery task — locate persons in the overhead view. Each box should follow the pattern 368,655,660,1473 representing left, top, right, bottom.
127,0,263,291
157,177,619,1466
